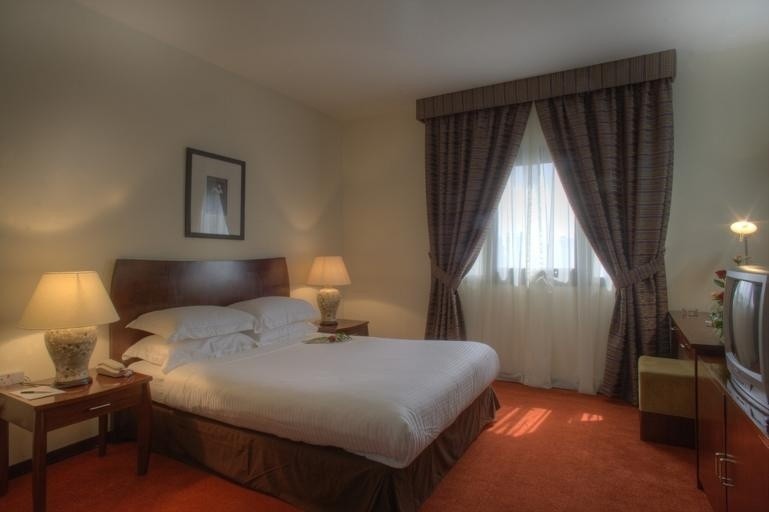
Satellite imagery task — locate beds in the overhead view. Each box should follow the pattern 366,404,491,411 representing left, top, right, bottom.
109,256,501,511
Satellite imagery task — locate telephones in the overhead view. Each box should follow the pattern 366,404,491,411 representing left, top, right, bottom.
96,358,129,378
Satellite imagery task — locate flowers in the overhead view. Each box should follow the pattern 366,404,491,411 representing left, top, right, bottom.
709,255,752,339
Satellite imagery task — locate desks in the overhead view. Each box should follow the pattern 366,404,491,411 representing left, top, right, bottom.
1,366,154,512
310,318,370,336
665,310,725,363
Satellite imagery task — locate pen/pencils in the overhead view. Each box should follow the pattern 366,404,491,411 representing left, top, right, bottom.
21,391,53,393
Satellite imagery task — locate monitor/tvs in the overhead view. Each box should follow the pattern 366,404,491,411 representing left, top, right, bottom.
723,266,769,439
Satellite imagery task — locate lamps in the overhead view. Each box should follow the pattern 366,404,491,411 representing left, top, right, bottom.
729,217,758,264
305,256,352,326
16,270,121,389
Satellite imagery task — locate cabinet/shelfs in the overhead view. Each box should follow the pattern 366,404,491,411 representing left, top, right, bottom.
695,355,769,512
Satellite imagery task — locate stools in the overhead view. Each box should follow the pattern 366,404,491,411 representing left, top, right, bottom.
637,355,694,448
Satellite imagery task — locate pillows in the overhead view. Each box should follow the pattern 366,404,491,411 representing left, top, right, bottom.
121,295,321,381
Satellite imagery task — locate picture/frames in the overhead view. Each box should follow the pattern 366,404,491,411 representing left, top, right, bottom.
185,146,246,241
